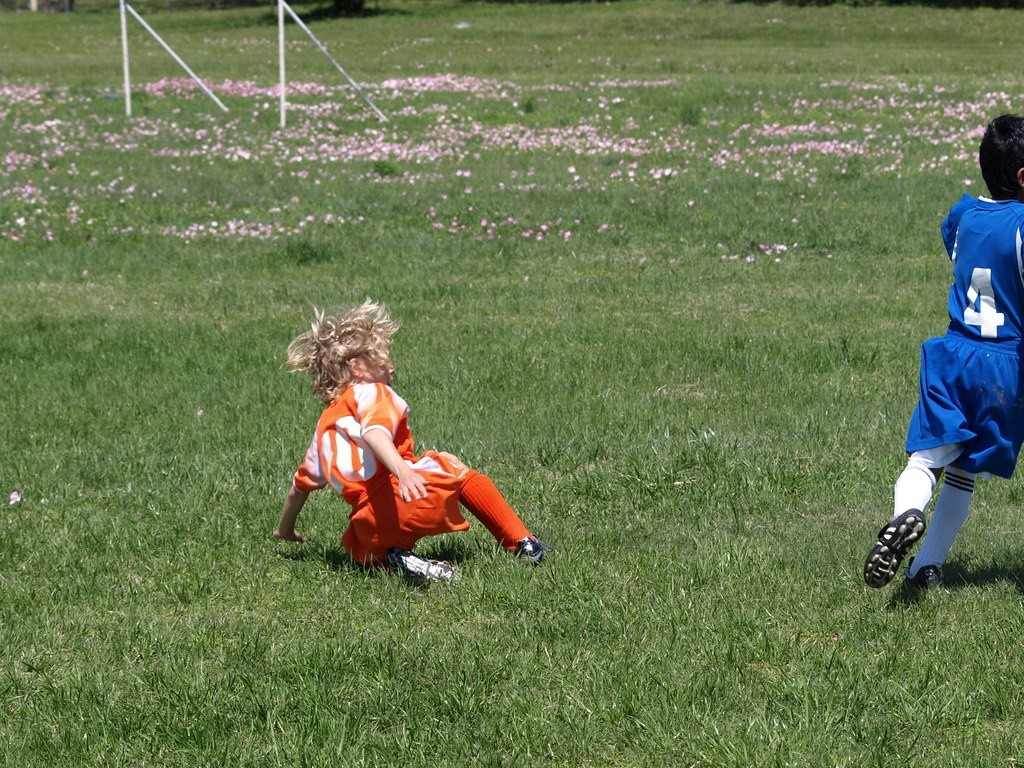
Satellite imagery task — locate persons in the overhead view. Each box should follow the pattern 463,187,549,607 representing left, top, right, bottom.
273,295,553,588
864,114,1024,608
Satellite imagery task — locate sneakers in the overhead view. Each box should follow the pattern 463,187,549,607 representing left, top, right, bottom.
864,508,926,588
514,535,551,568
895,556,943,602
386,547,461,586
